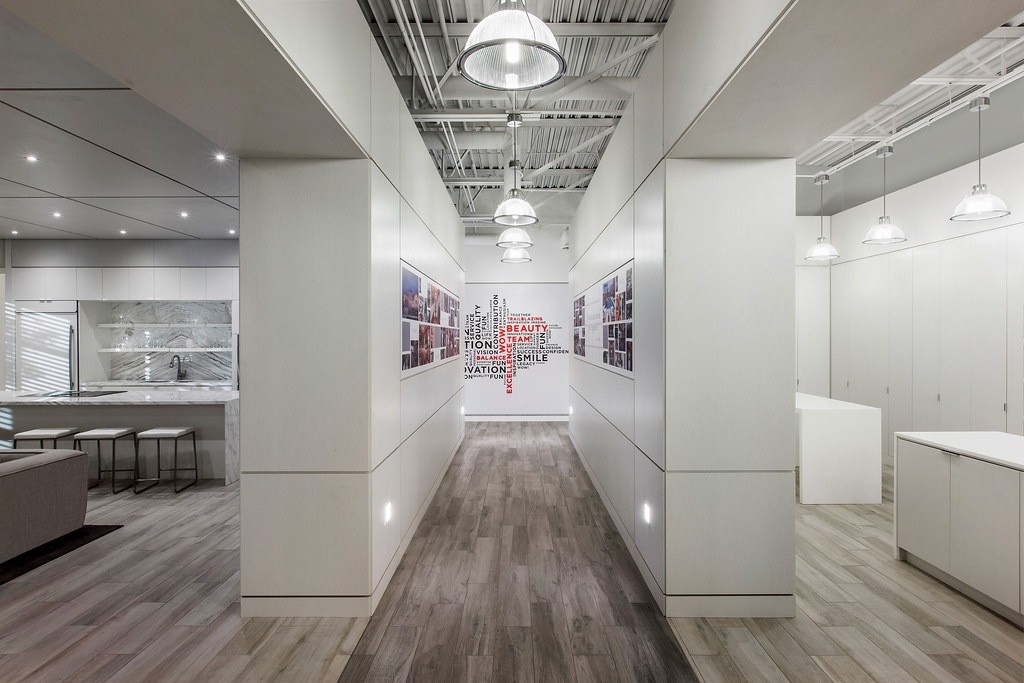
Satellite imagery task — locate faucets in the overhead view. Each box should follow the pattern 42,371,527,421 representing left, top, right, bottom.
169,354,187,380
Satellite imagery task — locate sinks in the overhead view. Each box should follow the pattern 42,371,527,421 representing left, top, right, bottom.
140,381,194,384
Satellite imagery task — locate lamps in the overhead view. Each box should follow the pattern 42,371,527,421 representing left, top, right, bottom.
491,91,539,265
861,146,908,246
560,225,570,250
804,175,840,261
457,0,567,92
950,96,1012,222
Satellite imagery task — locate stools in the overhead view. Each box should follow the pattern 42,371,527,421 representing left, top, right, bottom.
133,426,198,495
13,427,80,450
73,427,140,495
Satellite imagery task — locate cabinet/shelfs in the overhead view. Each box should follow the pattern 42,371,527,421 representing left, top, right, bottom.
893,430,1024,633
794,390,882,504
78,300,235,386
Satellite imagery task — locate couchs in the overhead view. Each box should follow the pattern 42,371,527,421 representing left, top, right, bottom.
0,448,88,565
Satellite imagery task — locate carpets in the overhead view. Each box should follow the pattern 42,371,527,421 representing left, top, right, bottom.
0,525,123,588
